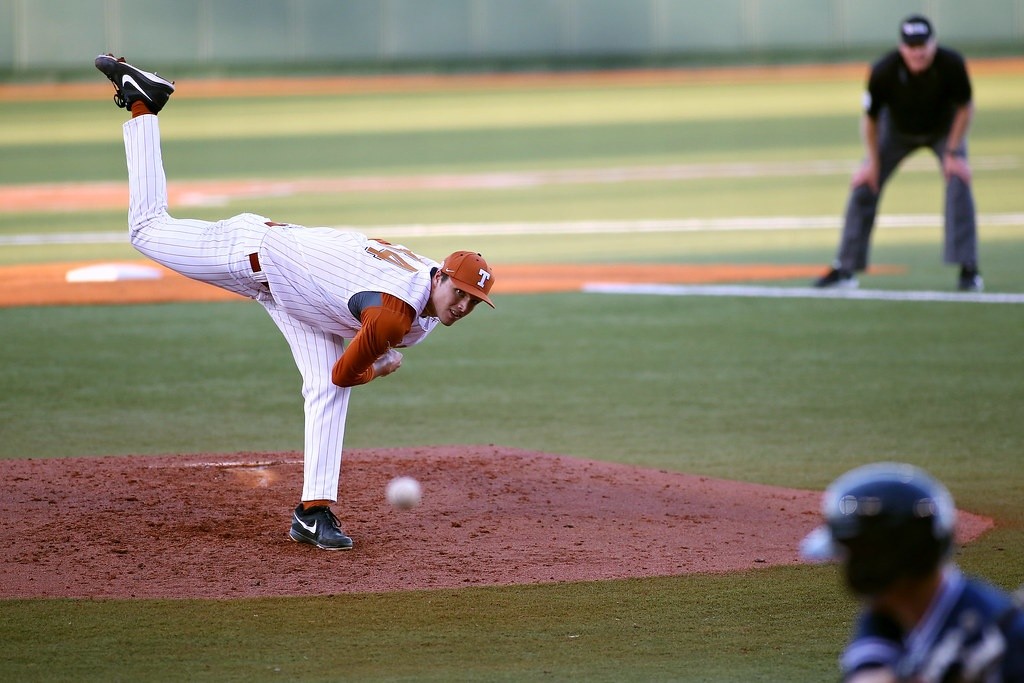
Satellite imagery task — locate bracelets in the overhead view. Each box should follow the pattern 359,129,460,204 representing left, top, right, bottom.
945,150,964,156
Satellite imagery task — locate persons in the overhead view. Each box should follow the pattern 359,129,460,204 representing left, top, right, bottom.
816,15,983,292
798,462,1024,682
94,53,496,549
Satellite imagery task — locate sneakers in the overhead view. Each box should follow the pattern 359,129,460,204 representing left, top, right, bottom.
95,55,176,115
289,504,353,551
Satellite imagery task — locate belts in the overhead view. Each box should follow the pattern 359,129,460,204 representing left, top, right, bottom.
249,222,289,290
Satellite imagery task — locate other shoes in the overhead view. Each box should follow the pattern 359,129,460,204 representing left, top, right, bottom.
959,270,984,291
813,267,859,289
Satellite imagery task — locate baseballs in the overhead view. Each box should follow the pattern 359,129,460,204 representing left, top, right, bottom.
385,477,423,508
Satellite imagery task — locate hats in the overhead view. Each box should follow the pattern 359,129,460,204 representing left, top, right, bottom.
438,250,495,309
899,14,935,46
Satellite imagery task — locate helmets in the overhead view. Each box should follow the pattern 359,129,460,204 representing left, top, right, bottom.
798,460,959,556
